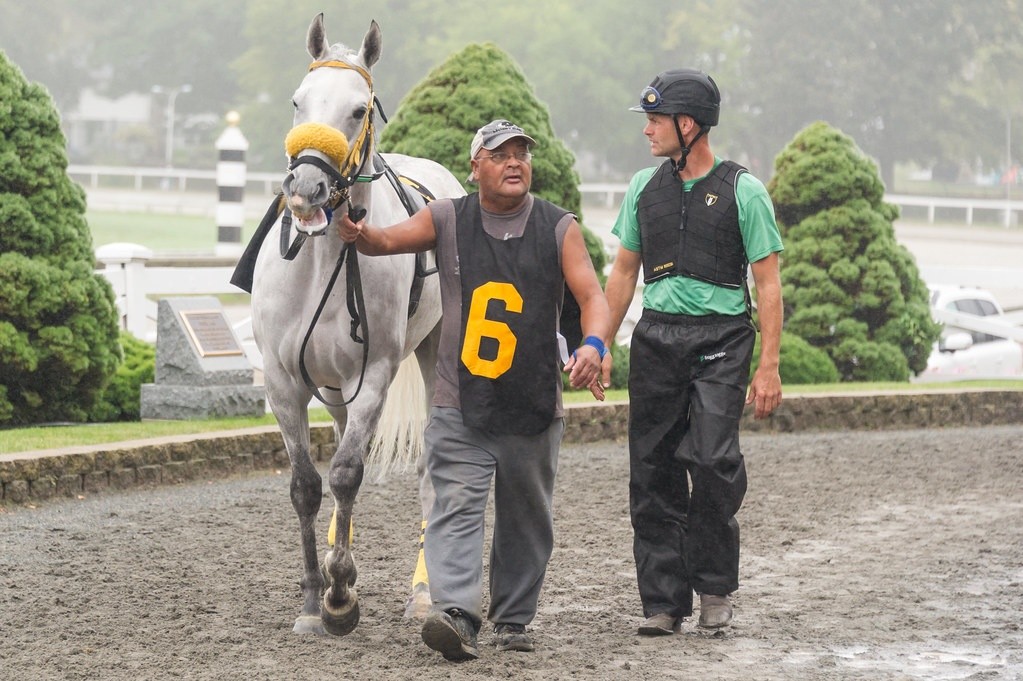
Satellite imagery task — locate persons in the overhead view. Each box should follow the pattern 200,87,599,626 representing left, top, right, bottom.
589,66,788,636
338,115,609,665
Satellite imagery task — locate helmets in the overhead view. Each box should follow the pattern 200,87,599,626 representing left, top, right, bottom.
628,68,724,127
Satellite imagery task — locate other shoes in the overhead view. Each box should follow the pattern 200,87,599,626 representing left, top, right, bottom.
492,623,536,651
421,610,483,659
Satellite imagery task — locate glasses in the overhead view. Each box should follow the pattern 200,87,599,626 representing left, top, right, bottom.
476,153,538,163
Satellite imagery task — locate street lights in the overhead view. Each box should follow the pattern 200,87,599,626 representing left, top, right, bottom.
151,85,192,170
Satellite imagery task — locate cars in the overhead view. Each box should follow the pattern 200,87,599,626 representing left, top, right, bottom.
919,287,1023,376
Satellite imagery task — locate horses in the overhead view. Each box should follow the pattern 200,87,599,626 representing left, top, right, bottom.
250,12,470,635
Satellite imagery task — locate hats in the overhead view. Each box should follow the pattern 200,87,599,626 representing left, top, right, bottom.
471,120,537,159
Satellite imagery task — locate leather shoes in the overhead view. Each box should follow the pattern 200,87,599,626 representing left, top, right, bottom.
698,593,732,628
638,613,683,633
